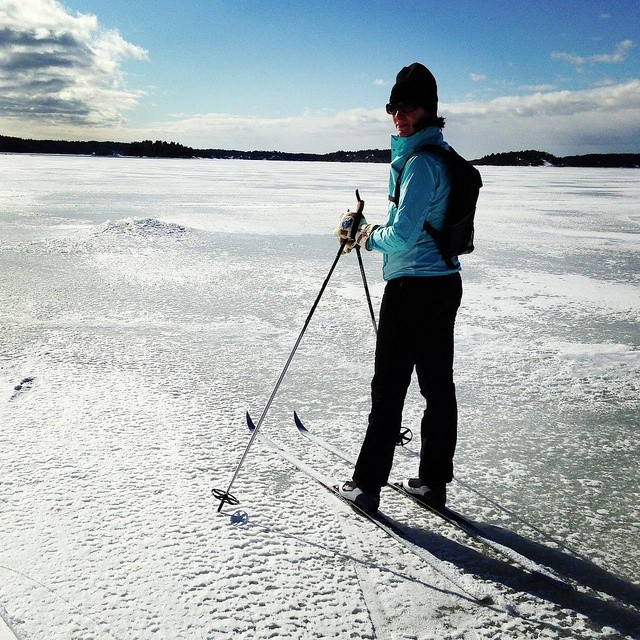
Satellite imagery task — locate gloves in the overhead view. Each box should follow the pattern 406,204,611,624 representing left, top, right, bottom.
338,209,380,232
338,229,371,255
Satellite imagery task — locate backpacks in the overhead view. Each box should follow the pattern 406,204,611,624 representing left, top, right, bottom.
394,144,482,269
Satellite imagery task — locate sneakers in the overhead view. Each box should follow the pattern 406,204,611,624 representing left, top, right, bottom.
402,477,446,510
338,481,381,515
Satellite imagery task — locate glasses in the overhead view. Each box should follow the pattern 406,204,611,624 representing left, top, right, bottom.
386,101,421,114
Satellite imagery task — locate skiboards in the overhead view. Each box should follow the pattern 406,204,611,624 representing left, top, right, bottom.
246,411,541,601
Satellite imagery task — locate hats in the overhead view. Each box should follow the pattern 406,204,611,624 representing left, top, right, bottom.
390,62,438,112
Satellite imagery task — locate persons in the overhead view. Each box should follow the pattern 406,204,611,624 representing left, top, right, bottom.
332,62,483,514
